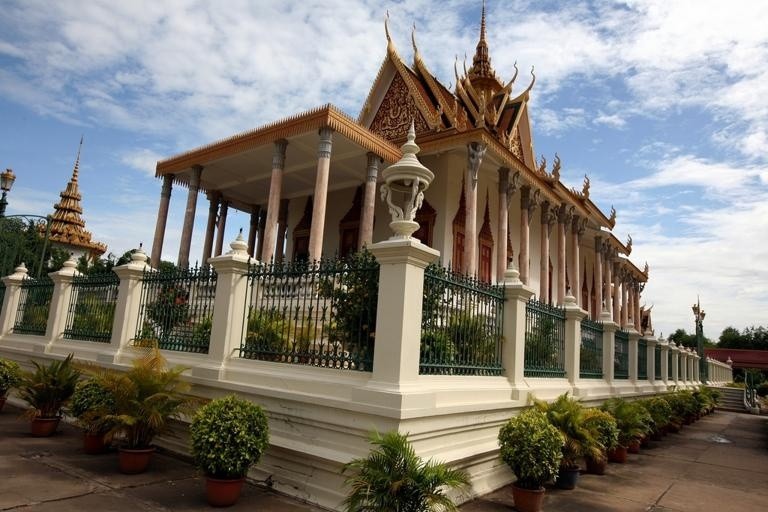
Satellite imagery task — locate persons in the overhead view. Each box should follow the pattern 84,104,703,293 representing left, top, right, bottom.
467,142,490,180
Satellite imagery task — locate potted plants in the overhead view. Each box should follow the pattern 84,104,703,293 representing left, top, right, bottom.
72,378,118,456
78,338,204,474
14,352,85,436
498,385,724,512
191,395,270,507
0,359,23,415
